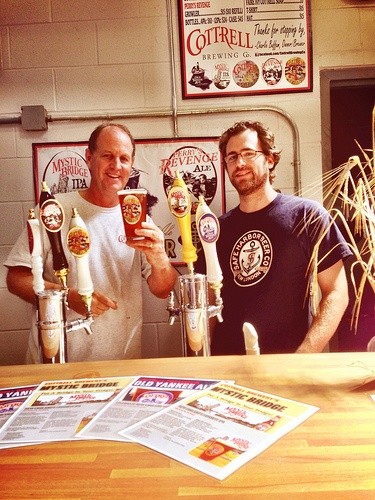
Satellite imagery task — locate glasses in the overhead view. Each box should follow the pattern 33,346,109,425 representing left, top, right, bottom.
223,150,265,163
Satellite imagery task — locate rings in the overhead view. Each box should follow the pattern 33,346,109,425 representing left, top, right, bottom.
150,242,154,249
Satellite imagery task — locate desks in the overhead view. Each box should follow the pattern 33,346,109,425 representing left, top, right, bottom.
0,352,375,500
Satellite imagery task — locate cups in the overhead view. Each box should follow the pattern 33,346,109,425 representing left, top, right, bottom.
118,189,147,241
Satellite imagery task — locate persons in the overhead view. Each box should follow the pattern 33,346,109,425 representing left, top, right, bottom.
3,124,176,364
192,120,353,357
